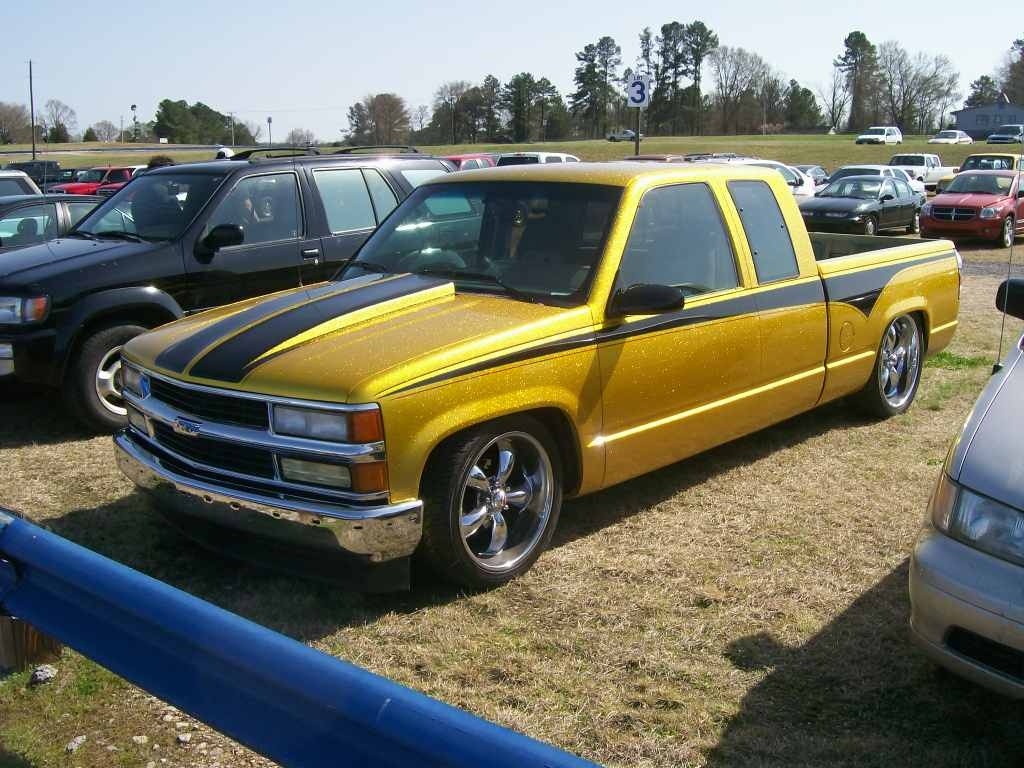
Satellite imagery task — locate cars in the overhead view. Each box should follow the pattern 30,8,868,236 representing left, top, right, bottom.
919,169,1024,249
928,130,974,145
0,149,1024,432
985,123,1024,144
799,174,921,235
856,126,904,146
907,276,1024,698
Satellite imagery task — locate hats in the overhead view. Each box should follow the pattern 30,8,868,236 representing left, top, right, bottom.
217,146,235,158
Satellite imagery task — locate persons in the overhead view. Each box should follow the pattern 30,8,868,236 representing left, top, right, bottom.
131,156,186,233
212,147,260,225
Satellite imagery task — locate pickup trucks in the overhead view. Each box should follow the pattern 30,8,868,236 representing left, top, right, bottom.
606,130,643,143
112,161,964,591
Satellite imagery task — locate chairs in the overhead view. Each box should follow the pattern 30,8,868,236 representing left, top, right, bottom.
642,224,716,288
509,213,578,287
